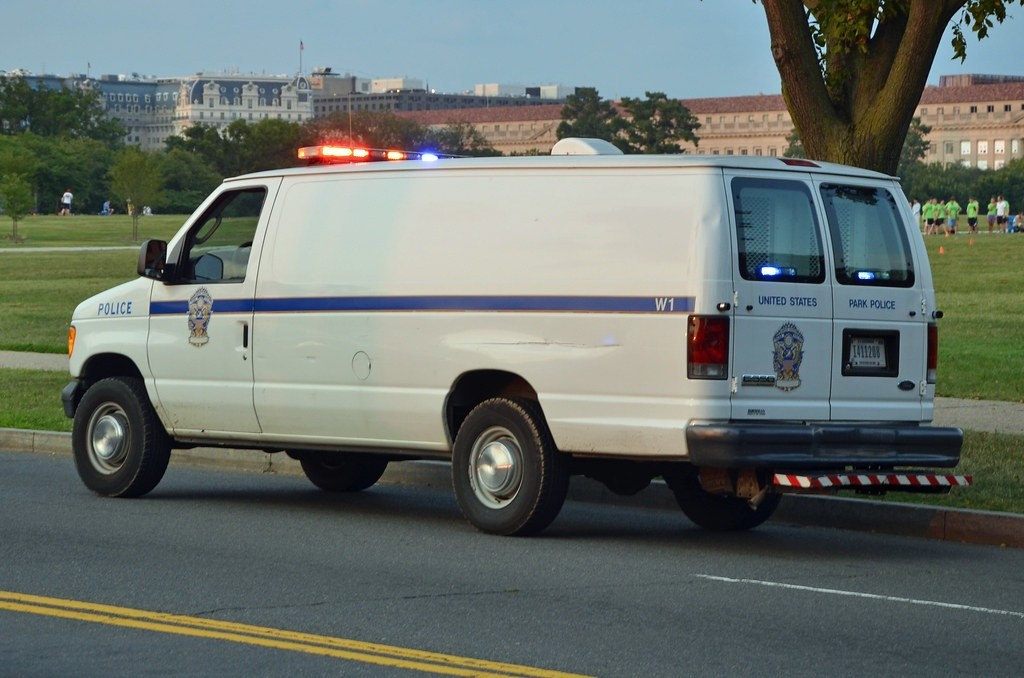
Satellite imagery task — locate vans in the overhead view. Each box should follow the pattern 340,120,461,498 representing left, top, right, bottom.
60,138,973,538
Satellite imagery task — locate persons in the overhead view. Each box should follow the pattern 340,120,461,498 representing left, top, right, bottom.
60,188,75,215
101,200,150,217
910,196,1024,235
